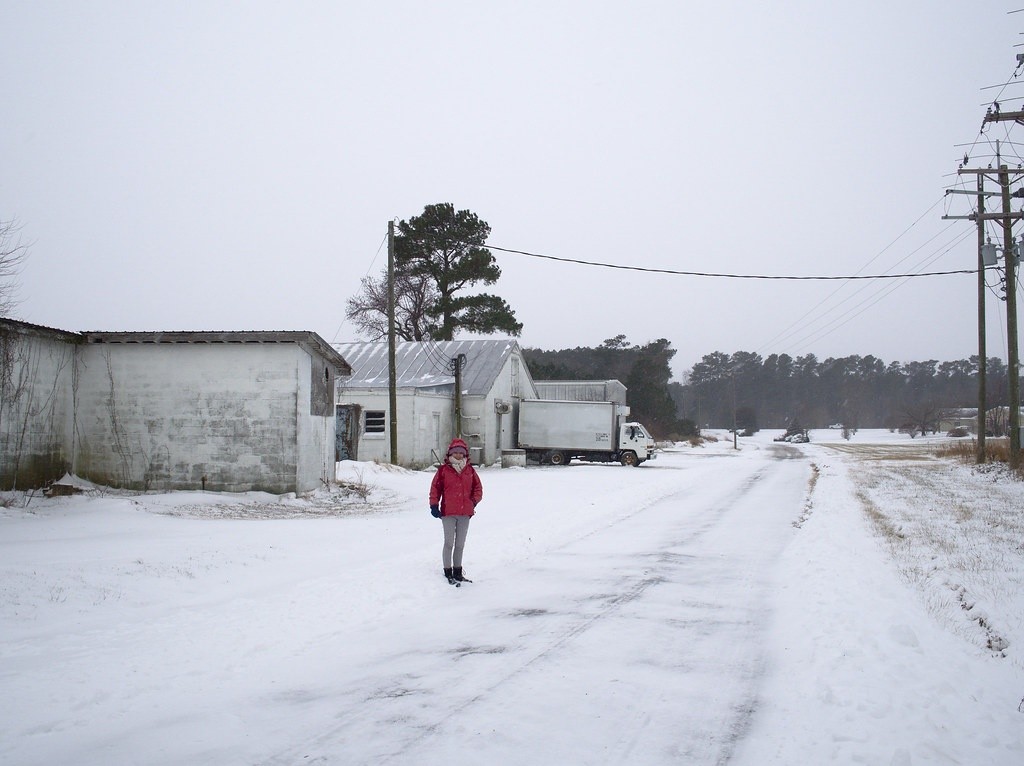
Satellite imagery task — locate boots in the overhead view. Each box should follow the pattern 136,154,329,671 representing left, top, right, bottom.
444,566,472,587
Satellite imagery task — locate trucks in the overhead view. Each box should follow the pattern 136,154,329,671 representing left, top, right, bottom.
517,396,655,468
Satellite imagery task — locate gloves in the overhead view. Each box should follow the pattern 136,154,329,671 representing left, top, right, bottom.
430,504,442,519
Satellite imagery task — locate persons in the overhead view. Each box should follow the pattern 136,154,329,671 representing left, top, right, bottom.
429,439,483,589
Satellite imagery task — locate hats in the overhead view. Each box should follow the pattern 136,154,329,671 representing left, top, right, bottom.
448,447,466,456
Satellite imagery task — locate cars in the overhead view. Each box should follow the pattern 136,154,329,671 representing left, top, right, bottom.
828,423,844,429
